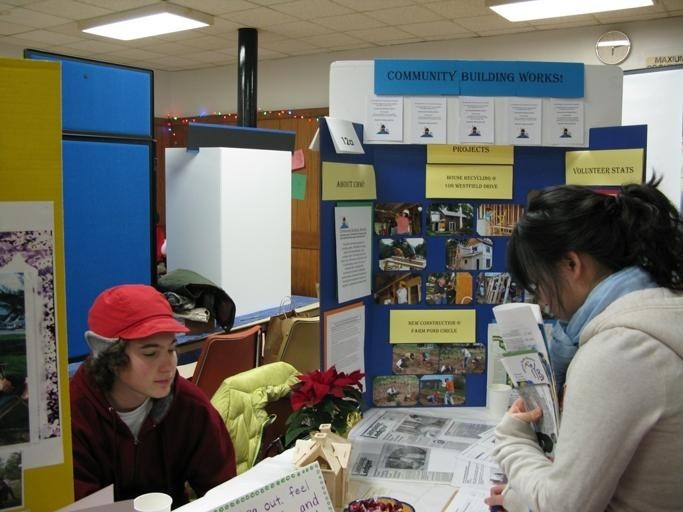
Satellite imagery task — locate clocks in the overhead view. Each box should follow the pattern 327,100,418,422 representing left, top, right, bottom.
595,30,631,65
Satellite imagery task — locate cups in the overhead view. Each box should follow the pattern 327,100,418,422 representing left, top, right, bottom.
488,384,512,417
133,493,173,512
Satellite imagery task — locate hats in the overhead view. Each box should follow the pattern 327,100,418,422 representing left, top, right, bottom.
85,283,190,358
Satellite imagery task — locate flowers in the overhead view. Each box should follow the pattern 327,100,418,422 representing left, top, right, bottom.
282,365,366,447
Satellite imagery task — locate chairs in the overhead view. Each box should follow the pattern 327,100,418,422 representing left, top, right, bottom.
191,325,263,400
279,317,321,373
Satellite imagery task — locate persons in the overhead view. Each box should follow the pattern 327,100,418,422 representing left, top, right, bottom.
375,204,496,407
67,282,238,510
339,217,349,228
481,166,683,511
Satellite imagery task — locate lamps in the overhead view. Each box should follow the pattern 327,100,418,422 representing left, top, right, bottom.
487,0,654,23
80,3,214,41
